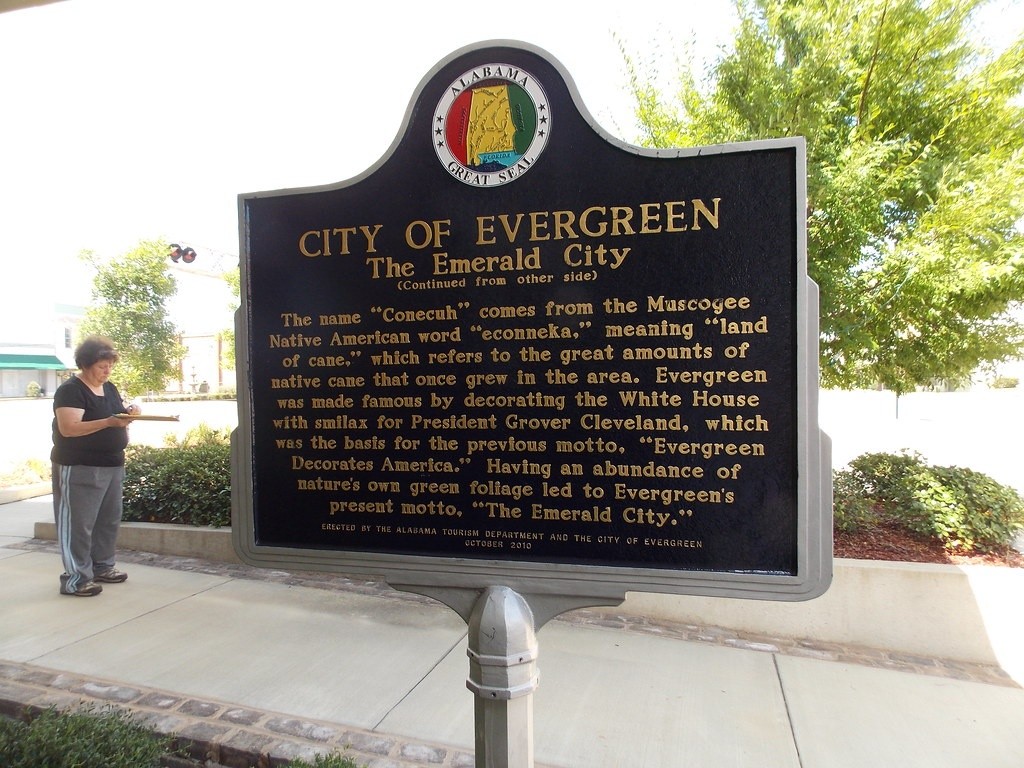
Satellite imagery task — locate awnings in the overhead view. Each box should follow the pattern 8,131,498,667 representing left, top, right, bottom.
0,354,67,371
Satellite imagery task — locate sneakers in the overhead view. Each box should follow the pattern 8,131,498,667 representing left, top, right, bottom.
91,567,128,583
60,572,103,596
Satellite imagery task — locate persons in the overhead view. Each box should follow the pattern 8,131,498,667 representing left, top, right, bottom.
50,333,143,597
